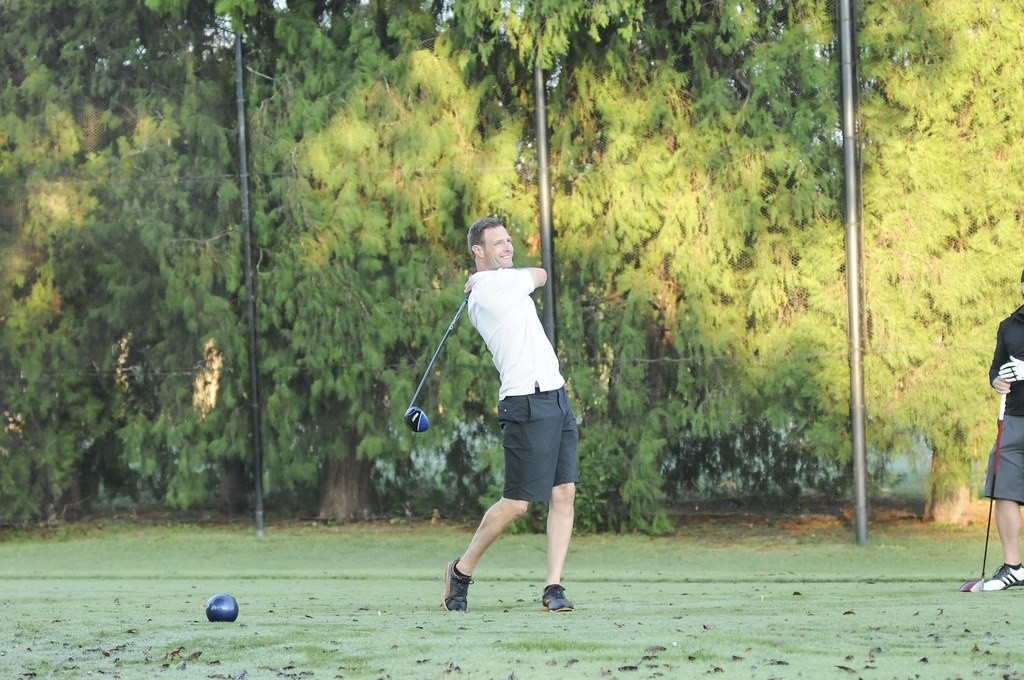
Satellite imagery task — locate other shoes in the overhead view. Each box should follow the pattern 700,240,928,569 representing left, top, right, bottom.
441,556,476,612
981,563,1024,591
541,584,575,611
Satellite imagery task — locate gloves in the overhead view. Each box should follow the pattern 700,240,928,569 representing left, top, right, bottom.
996,355,1024,383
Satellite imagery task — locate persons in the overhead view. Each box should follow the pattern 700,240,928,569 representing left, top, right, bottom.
983,270,1024,591
444,218,580,613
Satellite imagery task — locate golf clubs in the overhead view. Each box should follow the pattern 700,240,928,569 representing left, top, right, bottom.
405,290,470,432
959,393,1006,593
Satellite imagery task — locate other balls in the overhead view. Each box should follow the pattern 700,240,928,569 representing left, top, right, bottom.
205,593,239,623
